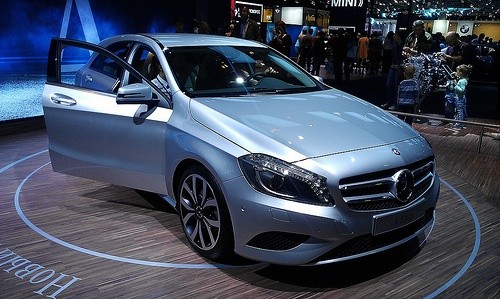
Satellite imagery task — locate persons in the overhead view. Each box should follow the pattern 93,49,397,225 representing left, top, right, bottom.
236,51,280,86
295,26,402,73
232,6,259,41
268,20,292,57
404,19,500,77
151,55,172,99
396,65,418,126
452,64,469,129
174,18,210,32
380,31,405,110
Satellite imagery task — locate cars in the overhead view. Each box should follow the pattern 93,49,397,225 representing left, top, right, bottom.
40,33,442,269
469,43,499,84
